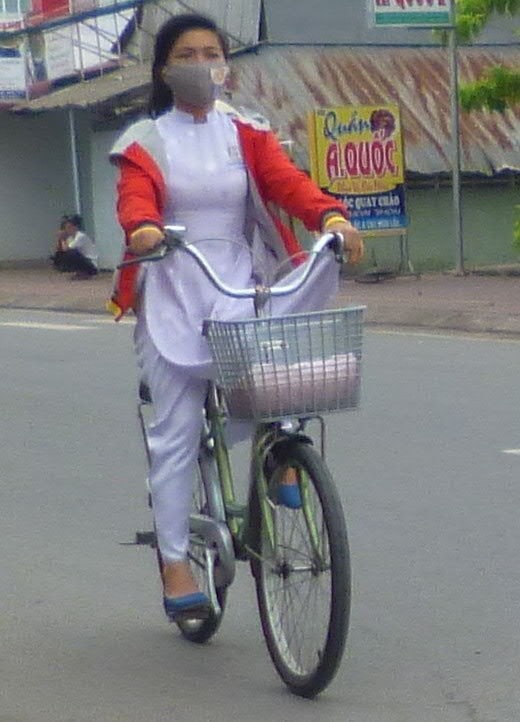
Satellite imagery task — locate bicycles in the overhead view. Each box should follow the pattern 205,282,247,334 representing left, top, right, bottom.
118,224,369,698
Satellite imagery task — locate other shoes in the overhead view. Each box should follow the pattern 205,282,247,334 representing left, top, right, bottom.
262,463,302,509
164,591,210,614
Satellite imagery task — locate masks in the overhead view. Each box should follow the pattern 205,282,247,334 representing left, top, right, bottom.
163,61,228,106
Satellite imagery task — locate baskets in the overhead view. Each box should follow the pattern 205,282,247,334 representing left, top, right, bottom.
200,304,368,424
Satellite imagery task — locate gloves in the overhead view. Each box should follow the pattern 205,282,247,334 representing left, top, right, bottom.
321,211,364,264
130,223,165,256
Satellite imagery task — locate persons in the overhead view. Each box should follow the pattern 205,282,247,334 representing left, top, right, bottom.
60,214,98,281
52,216,81,272
105,13,365,616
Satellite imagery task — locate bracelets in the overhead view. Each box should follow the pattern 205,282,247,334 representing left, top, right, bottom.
128,227,159,240
321,216,348,233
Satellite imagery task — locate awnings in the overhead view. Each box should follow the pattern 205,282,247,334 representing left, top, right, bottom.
9,63,152,114
